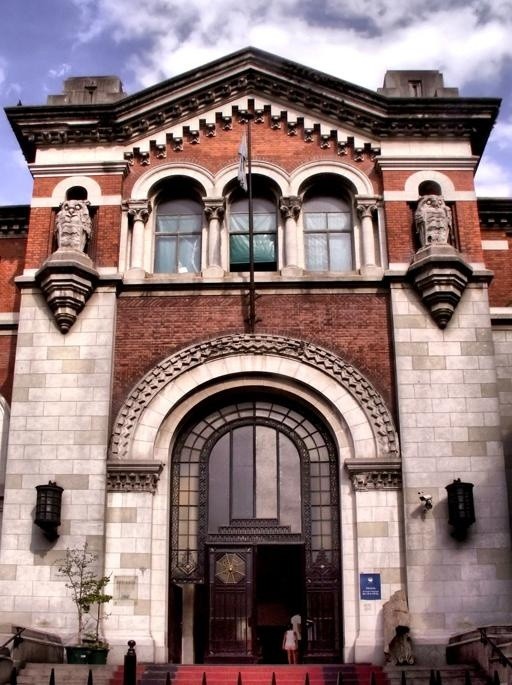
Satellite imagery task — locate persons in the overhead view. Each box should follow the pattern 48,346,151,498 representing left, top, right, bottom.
280,623,299,665
291,609,301,664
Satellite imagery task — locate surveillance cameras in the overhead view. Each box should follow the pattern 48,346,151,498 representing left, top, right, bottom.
419,494,432,501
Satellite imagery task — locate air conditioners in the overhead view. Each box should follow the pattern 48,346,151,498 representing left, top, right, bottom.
55,539,91,664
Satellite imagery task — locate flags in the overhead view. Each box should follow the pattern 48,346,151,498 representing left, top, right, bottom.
233,131,249,193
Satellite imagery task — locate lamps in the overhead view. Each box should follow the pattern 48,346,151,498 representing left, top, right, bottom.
445,478,476,542
34,481,63,542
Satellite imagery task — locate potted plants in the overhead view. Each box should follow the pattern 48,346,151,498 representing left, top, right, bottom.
78,570,112,665
